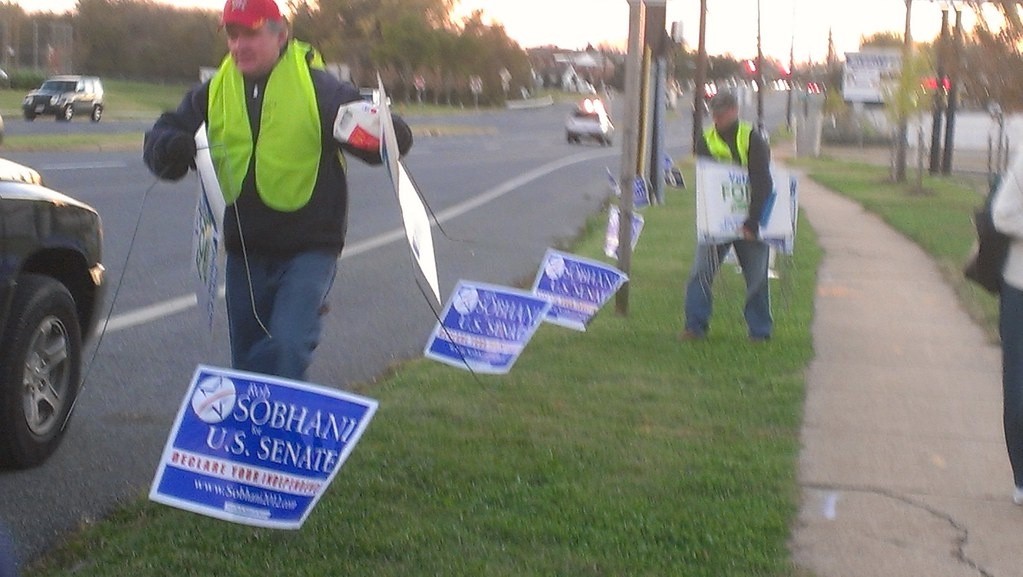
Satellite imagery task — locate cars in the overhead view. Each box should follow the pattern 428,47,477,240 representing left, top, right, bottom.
564,110,614,148
0,156,110,470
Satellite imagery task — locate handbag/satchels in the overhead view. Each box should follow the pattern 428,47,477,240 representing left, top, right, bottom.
964,175,1010,293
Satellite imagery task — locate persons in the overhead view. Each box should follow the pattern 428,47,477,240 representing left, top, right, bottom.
681,92,774,340
143,0,413,382
990,154,1023,505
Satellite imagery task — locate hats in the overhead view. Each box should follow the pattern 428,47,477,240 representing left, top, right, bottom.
216,0,282,33
711,87,738,111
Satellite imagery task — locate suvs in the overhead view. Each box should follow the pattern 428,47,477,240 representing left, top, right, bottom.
22,74,105,122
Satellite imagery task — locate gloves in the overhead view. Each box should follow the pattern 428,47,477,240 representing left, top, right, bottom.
167,132,197,171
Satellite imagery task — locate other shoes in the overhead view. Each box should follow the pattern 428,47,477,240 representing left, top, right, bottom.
1014,486,1022,506
676,328,695,342
748,333,768,346
